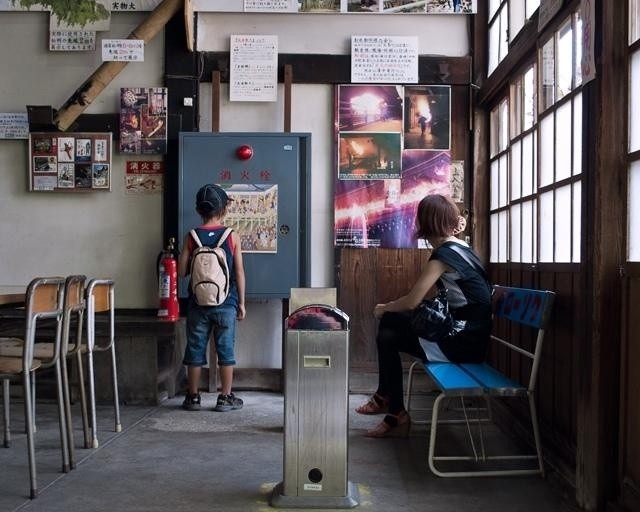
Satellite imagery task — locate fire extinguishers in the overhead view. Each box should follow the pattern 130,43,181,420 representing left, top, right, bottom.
156,238,179,322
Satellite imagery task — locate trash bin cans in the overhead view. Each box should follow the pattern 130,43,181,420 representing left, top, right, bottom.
271,304,359,508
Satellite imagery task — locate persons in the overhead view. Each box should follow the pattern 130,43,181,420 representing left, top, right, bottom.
178,184,246,412
355,194,493,439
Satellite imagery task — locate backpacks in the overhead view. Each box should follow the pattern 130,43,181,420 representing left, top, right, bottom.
189,227,233,307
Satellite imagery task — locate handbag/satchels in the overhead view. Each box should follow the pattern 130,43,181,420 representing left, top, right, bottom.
412,296,455,342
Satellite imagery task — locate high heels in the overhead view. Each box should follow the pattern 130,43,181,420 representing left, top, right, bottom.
362,411,411,437
355,392,403,415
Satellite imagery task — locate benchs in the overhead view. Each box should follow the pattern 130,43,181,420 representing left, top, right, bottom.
406,285,556,479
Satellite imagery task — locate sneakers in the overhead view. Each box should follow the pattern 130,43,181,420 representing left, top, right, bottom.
215,392,243,411
183,388,200,410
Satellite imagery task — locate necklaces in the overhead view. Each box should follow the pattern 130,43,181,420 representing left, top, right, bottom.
440,236,454,244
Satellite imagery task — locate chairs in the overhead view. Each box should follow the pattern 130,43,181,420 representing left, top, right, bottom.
0,273,122,499
